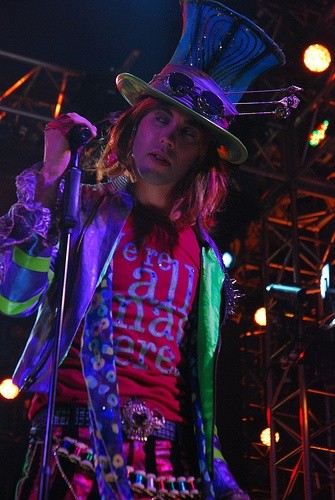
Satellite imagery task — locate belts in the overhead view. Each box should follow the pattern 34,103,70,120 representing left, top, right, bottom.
31,403,186,442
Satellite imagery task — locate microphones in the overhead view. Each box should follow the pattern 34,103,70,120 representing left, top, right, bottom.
70,125,93,145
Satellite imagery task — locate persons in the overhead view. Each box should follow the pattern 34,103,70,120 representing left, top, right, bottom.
2,87,247,496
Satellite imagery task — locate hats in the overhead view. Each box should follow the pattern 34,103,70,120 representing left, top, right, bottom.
115,0,303,165
